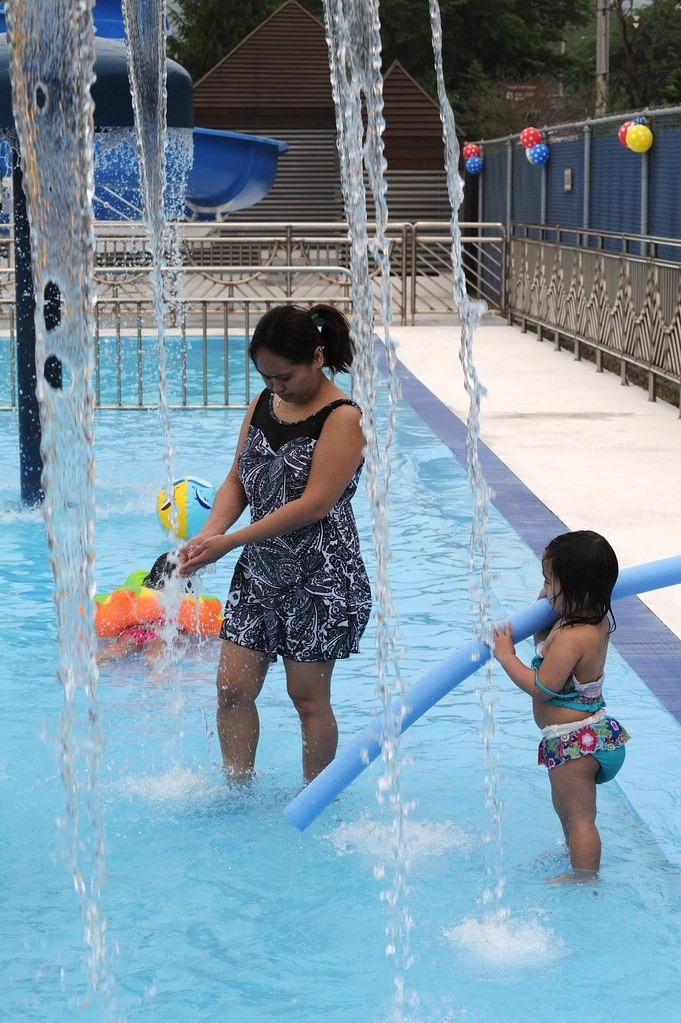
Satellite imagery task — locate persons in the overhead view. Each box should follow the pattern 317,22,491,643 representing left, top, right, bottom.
479,528,629,886
177,303,373,810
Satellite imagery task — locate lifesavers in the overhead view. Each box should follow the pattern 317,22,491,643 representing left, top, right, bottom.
280,554,681,834
93,570,225,638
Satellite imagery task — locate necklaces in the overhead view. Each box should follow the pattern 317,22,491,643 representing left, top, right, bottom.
276,374,327,413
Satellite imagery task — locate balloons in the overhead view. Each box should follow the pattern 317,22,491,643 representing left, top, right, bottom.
520,127,550,166
462,144,483,175
618,117,653,153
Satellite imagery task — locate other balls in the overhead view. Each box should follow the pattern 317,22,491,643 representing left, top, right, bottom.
156,472,222,548
